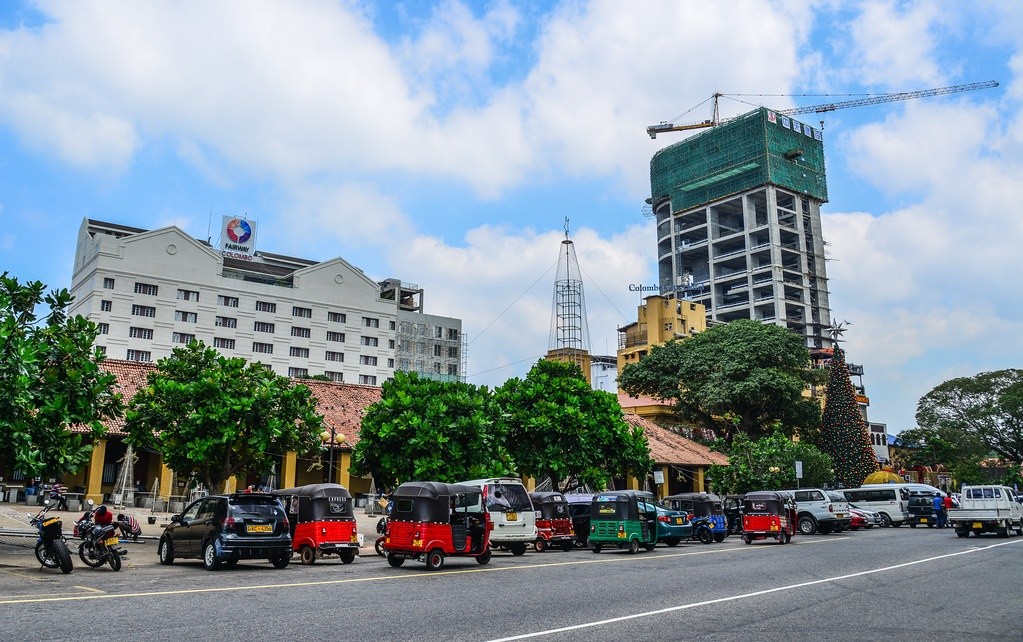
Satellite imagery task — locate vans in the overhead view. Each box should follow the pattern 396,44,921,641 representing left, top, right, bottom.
834,487,910,528
861,483,948,499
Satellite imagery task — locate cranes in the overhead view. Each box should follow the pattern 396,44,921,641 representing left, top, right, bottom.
646,79,999,139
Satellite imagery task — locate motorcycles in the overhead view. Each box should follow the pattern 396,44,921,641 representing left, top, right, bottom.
527,489,659,554
271,483,360,564
26,499,73,574
658,493,728,545
74,498,128,571
723,491,798,545
374,482,492,571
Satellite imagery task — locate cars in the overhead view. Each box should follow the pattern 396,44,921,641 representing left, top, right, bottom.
157,492,293,571
638,502,693,546
849,502,881,531
564,495,593,547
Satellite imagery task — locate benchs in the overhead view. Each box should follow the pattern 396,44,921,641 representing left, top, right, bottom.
44,491,59,511
26,494,38,506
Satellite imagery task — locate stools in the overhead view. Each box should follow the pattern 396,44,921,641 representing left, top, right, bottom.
151,501,167,512
68,499,80,512
145,498,155,508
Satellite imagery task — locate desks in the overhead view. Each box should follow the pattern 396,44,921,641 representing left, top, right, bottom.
134,492,159,508
62,492,85,510
5,483,23,503
163,494,187,514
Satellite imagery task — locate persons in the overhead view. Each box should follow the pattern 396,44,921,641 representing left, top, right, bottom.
385,485,393,514
94,506,112,527
117,514,142,539
291,496,299,515
932,491,955,529
243,486,263,493
73,511,93,537
50,482,67,510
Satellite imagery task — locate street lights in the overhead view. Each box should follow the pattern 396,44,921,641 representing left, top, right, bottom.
320,426,345,483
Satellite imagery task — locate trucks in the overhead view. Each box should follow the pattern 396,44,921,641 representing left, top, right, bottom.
946,485,1023,537
781,489,847,535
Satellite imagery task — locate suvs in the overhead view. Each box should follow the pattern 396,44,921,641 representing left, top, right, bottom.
450,477,539,556
906,490,959,528
